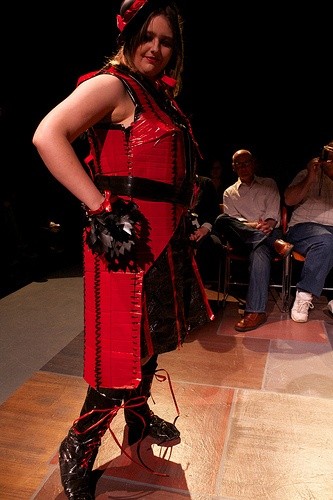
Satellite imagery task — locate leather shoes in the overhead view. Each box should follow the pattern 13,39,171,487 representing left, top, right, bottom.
273,239,293,257
235,312,266,331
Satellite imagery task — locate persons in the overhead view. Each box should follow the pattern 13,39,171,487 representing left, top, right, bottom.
33,0,204,500
191,177,215,287
214,150,294,331
285,143,333,323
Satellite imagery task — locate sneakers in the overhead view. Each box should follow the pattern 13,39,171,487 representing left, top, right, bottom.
328,300,333,313
290,290,314,322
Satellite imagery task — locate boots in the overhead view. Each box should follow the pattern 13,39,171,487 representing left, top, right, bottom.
59,387,122,500
122,354,179,441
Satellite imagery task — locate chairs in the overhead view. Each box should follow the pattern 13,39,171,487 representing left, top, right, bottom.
288,204,333,313
206,205,289,313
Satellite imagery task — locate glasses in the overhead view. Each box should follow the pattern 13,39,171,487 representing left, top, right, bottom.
233,161,251,167
